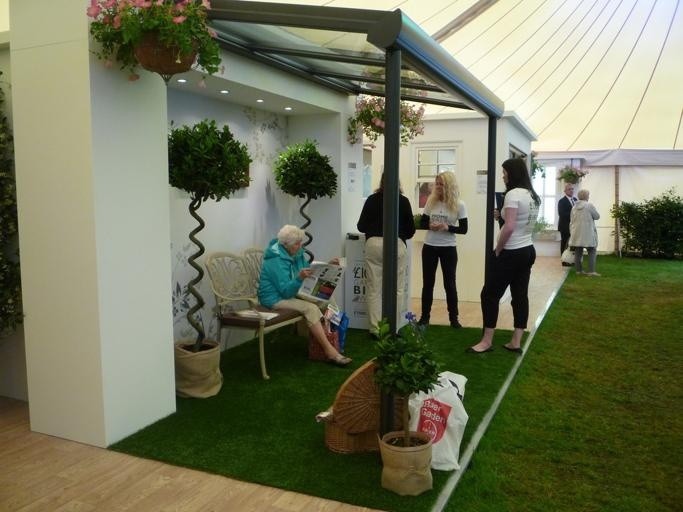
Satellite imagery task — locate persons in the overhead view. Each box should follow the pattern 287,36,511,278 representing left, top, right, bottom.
356,172,415,340
417,170,469,328
256,224,354,367
464,158,542,354
569,189,601,275
557,183,578,267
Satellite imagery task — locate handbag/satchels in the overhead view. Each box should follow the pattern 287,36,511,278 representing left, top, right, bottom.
305,327,340,362
561,245,584,267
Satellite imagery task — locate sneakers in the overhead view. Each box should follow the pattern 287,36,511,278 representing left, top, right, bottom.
326,351,353,366
501,343,523,354
416,315,461,330
464,344,494,353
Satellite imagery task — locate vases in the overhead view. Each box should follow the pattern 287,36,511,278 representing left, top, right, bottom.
132,32,197,81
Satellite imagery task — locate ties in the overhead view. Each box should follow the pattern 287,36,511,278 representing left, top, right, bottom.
570,198,575,206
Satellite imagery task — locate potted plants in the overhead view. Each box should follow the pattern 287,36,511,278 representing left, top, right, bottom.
169,117,252,401
274,139,338,340
86,0,227,83
372,313,446,496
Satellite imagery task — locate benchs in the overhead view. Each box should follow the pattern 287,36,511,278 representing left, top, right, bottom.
206,248,338,379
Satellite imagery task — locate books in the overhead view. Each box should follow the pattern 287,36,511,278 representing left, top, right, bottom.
237,309,279,320
297,257,346,301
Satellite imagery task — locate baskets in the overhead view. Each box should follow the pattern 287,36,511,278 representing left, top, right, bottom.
322,354,411,456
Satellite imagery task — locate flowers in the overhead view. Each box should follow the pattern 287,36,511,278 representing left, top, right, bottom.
558,165,586,184
348,96,425,149
